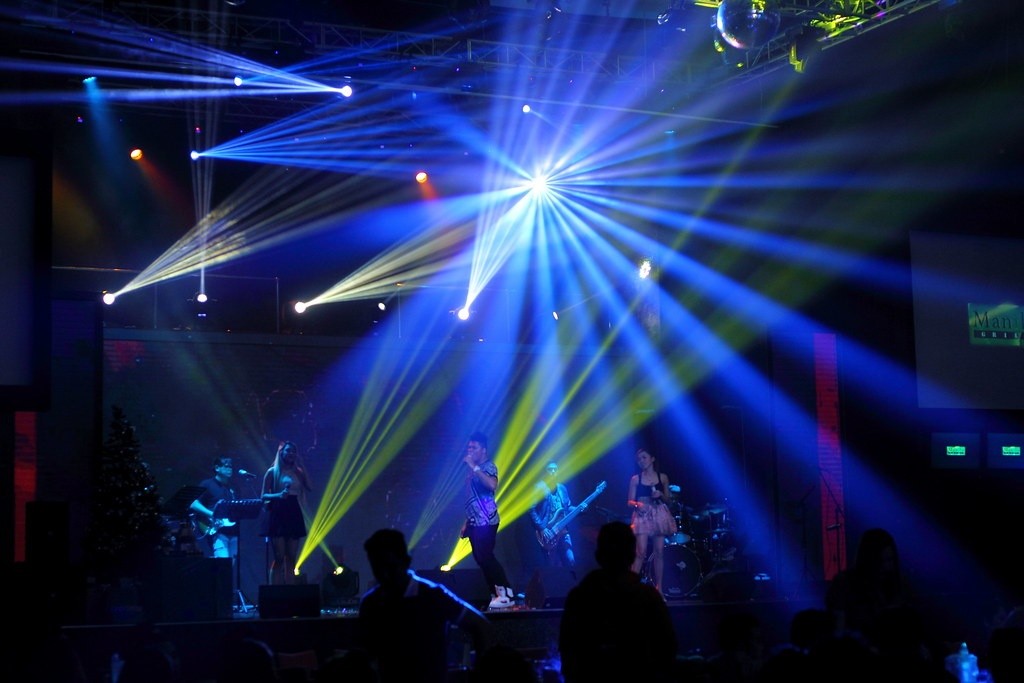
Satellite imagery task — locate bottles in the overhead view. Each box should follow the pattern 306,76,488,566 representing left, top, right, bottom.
956,643,978,683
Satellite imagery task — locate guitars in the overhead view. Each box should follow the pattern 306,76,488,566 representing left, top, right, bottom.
644,541,702,602
532,479,611,552
197,507,235,537
663,510,696,545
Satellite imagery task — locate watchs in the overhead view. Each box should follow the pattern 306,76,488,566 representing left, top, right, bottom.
473,464,480,472
635,502,638,508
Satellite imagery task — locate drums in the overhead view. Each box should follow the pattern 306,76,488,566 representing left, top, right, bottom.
693,508,733,531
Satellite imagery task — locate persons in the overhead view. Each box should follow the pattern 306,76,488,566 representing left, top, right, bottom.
628,448,677,603
528,461,589,607
458,432,515,608
115,620,177,683
261,441,316,586
307,529,537,683
687,532,1024,683
559,522,679,683
216,621,278,683
189,457,241,598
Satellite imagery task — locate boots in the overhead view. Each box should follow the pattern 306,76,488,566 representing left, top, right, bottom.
488,585,515,609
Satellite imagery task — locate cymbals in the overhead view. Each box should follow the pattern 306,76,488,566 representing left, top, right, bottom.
677,492,702,510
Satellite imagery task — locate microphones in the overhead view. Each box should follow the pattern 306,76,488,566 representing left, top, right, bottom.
463,455,472,466
650,482,656,492
284,482,289,491
238,469,256,479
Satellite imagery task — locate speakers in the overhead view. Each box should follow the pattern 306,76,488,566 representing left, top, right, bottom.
256,583,321,620
